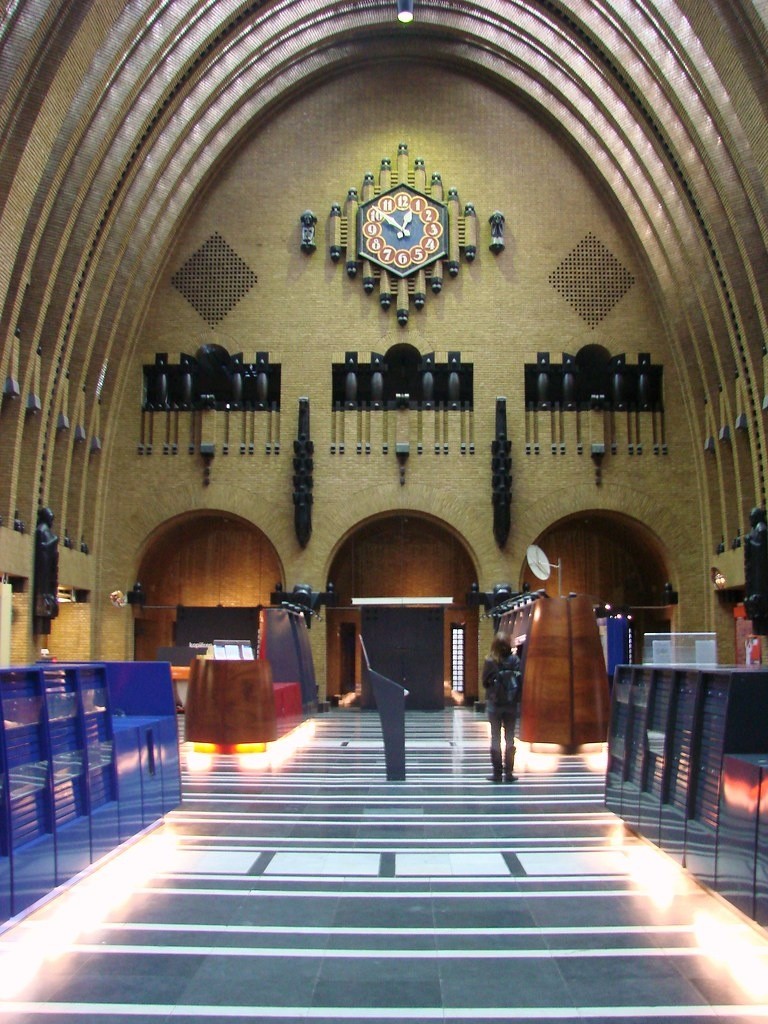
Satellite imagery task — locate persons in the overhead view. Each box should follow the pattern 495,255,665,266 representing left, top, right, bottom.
482,631,522,782
743,508,768,635
33,508,59,633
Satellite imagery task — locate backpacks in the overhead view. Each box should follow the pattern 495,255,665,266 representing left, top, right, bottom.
487,653,521,708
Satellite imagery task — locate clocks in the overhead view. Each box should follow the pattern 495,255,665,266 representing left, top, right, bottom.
358,183,448,279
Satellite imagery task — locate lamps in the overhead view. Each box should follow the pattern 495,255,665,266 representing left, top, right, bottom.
499,589,550,614
711,565,725,589
396,0,414,23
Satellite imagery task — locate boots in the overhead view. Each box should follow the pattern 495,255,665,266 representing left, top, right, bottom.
504,746,518,781
486,748,503,781
